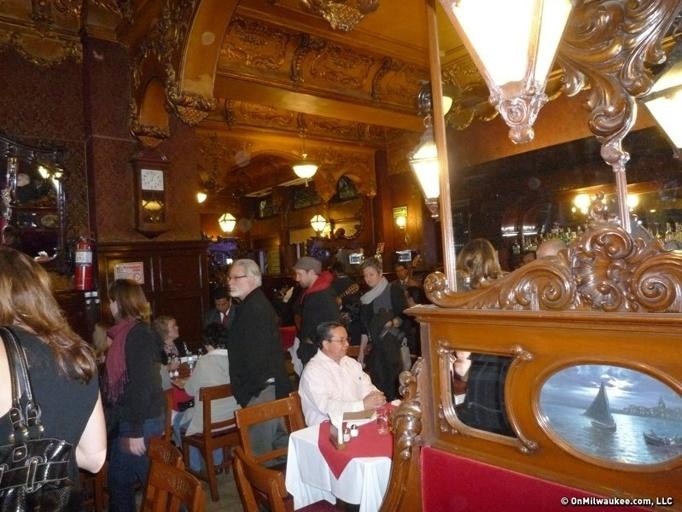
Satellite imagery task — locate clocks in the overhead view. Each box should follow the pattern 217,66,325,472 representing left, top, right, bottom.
129,144,173,240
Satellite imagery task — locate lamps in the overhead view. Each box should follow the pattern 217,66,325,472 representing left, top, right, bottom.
408,82,442,222
310,213,328,234
292,118,320,188
634,25,682,164
394,206,408,232
218,201,236,234
437,1,577,145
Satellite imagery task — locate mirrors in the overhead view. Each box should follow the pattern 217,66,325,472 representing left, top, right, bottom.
1,133,68,274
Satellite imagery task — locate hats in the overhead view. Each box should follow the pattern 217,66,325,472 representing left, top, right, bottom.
290,256,322,275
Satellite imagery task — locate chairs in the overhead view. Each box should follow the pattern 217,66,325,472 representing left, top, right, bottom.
69,304,422,512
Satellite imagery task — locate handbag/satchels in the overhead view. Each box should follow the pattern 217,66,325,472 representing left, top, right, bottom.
0,430,78,512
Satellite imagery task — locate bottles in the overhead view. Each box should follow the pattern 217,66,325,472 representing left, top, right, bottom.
343,428,351,443
12,211,39,230
644,221,682,251
277,286,294,304
512,222,585,256
165,347,203,379
350,424,360,438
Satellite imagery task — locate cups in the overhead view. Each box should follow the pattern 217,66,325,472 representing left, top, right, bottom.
377,416,389,436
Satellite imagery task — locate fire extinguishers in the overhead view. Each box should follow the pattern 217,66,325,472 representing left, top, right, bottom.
72,234,95,292
